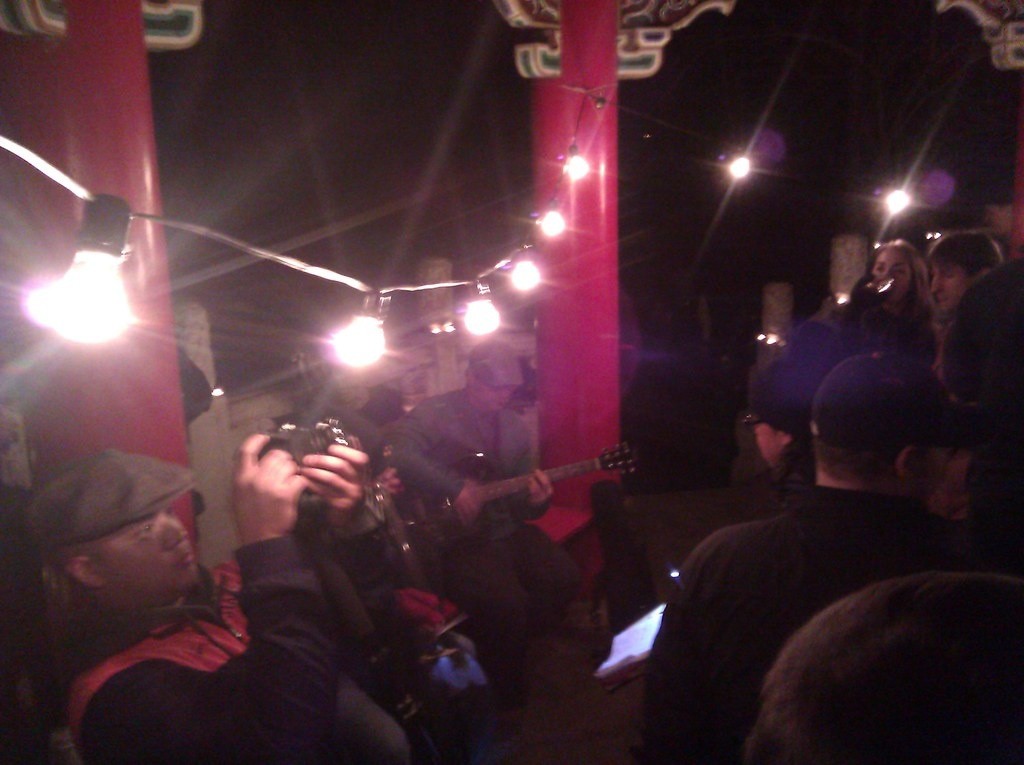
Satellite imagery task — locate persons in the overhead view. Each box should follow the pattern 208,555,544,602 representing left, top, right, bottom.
741,572,1024,765
732,226,1024,576
629,352,1005,765
385,342,585,714
22,433,442,765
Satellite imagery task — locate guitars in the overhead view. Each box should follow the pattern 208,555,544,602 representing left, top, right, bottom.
394,438,643,558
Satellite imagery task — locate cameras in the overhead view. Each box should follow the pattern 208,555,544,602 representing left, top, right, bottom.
258,418,352,494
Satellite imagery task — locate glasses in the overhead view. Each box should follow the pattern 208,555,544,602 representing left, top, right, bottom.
740,413,769,435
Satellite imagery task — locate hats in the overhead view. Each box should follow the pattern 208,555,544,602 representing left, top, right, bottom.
812,349,950,448
739,356,824,430
468,339,526,387
17,446,198,551
